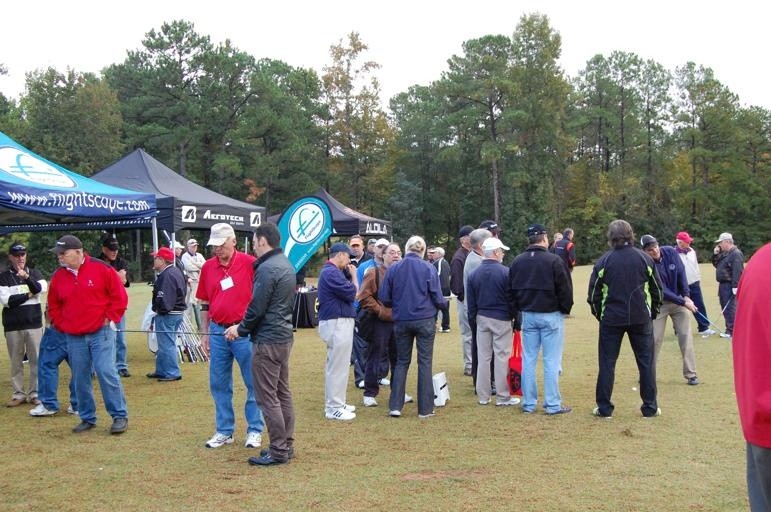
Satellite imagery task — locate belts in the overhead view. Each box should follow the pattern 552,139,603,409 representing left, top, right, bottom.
211,321,238,329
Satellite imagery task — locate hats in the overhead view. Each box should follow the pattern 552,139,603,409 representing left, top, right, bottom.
478,221,502,235
207,223,235,246
49,235,83,254
102,238,119,251
150,247,174,261
676,232,692,243
9,243,26,256
329,239,390,257
187,239,199,246
714,232,732,242
431,247,445,257
482,239,510,251
641,234,656,248
170,241,184,249
528,225,546,236
459,226,474,238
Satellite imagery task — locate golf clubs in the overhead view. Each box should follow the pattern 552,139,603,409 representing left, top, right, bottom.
109,320,239,338
701,294,734,338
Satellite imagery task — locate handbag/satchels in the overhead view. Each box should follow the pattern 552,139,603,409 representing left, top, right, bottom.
508,356,522,395
433,372,450,405
355,308,375,341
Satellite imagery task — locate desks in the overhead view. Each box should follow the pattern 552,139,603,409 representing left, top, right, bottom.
294,288,321,329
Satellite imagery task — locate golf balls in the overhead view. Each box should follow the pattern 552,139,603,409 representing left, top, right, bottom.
632,387,637,391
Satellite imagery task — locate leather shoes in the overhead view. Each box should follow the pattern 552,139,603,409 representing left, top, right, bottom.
160,376,181,381
147,373,164,378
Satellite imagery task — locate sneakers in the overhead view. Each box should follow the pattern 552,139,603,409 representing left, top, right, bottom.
325,404,356,420
6,397,59,416
357,377,436,418
464,369,471,375
480,383,519,406
249,448,293,465
654,408,661,416
720,333,730,338
687,377,697,384
593,408,601,416
547,407,571,415
111,417,129,432
73,423,95,432
206,432,234,449
121,369,130,376
245,433,262,446
699,330,715,334
68,405,78,415
